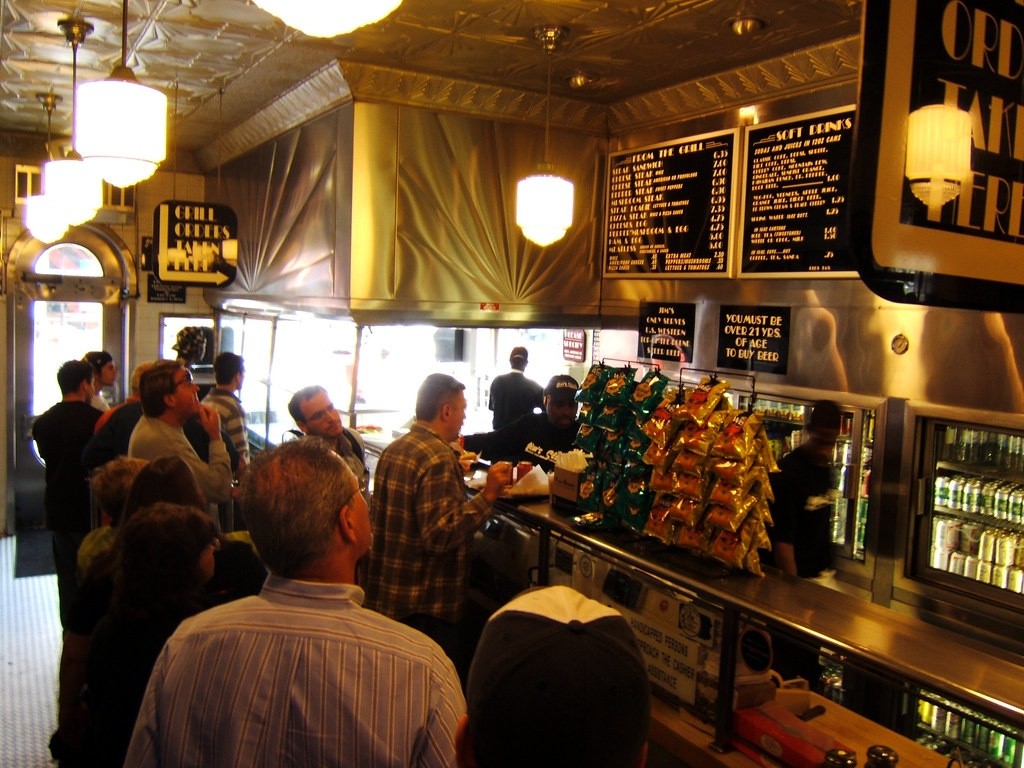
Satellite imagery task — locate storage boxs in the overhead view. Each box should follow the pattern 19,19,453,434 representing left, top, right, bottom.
551,466,588,504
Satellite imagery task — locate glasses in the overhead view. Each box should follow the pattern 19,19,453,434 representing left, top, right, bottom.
174,369,194,388
346,475,367,505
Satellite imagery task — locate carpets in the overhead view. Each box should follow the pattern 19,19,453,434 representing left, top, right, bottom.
14,490,46,533
13,530,58,579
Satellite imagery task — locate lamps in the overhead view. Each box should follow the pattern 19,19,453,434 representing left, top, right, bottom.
250,0,404,39
26,90,70,244
44,19,104,227
74,0,170,190
513,23,576,247
902,104,973,211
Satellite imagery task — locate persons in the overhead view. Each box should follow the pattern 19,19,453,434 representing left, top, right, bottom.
454,375,580,474
488,347,545,432
455,584,651,768
122,434,468,768
31,351,368,768
359,372,514,697
768,399,841,693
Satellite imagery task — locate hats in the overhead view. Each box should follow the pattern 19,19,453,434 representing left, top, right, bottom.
543,375,579,403
468,586,650,767
511,347,528,360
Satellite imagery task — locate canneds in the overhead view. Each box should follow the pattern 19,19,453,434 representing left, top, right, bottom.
498,461,512,484
791,411,874,547
915,689,1024,768
930,426,1024,594
516,461,532,483
821,662,843,703
